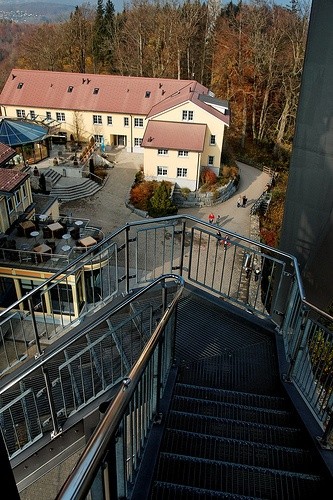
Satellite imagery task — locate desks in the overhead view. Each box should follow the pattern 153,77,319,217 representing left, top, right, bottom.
80,236,98,252
39,215,48,223
48,223,63,236
19,220,35,232
32,244,52,262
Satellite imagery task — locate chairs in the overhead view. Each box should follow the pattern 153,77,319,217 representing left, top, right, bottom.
69,226,100,252
42,219,68,239
17,220,40,239
31,240,56,262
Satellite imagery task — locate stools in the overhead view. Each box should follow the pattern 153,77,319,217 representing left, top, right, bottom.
75,221,84,226
30,231,39,241
62,234,71,245
61,246,71,263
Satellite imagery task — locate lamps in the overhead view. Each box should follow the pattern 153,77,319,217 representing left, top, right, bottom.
57,198,63,204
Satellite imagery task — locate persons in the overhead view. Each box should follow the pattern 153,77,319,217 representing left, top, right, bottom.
53,158,59,166
236,194,247,209
208,212,221,227
216,230,230,250
